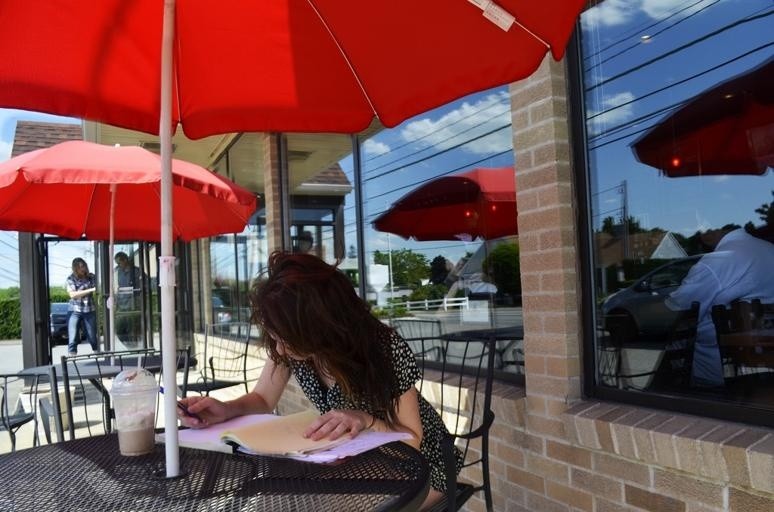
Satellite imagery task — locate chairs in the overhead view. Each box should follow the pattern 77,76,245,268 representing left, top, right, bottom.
603,297,773,396
597,325,621,389
388,319,446,365
0,321,251,453
401,334,495,511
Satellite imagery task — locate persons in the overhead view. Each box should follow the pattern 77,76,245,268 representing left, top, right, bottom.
113,251,150,351
67,255,100,360
652,190,774,396
176,249,466,512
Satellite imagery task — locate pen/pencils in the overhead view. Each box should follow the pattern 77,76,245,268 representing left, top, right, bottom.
159,386,204,423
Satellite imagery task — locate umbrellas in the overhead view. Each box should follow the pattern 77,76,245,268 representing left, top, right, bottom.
154,412,355,456
368,166,518,322
626,50,774,179
1,136,261,360
0,0,587,481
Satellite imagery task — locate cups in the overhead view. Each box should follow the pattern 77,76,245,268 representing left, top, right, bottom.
110,368,160,457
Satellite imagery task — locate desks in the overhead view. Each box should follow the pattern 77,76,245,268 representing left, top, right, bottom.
0,426,431,511
438,324,523,372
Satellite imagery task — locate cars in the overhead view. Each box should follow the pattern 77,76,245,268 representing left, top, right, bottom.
212,297,232,332
599,255,717,342
48,303,85,343
